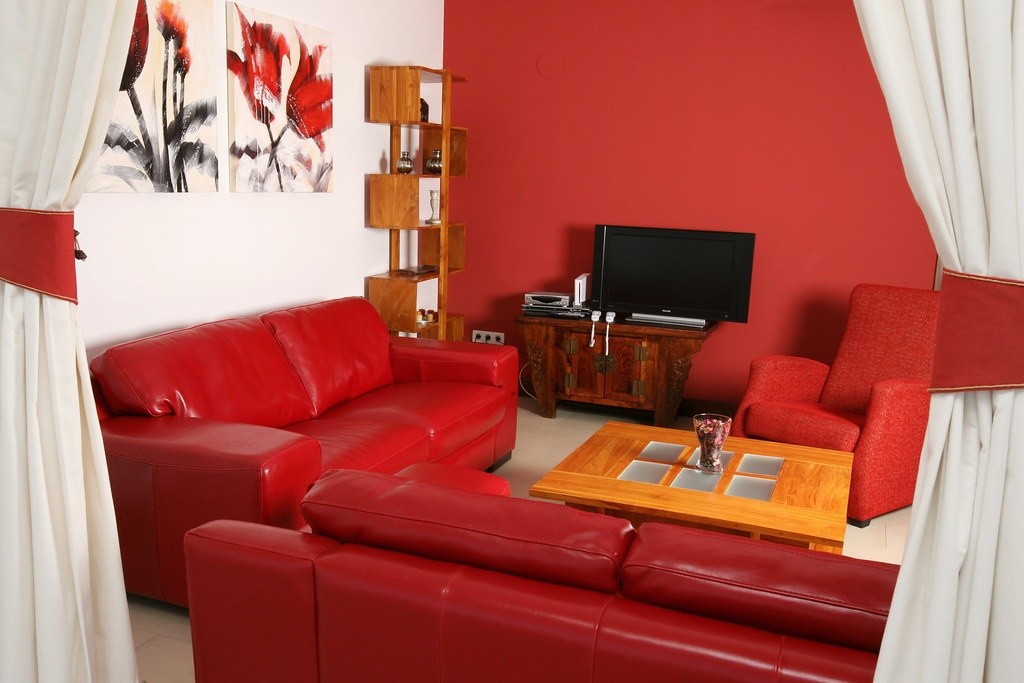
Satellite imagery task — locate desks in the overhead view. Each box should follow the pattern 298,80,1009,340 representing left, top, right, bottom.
527,421,858,554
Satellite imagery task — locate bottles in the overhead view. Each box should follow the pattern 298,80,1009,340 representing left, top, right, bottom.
693,413,732,475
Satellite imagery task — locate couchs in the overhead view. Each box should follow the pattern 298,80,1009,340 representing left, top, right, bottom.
181,463,899,683
722,280,943,523
88,293,522,613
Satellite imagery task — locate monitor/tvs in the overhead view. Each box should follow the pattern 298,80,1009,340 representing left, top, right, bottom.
590,223,756,329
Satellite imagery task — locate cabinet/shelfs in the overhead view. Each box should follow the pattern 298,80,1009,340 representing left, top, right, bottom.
363,61,471,346
512,303,724,425
